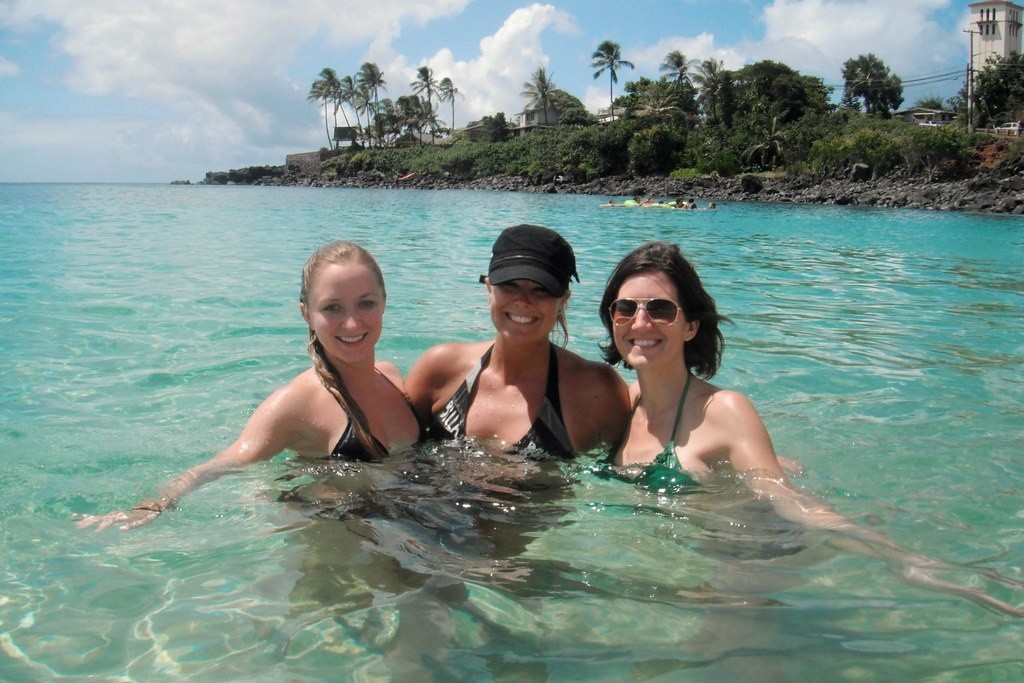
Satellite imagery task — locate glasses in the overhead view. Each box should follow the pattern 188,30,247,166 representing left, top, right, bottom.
606,297,684,327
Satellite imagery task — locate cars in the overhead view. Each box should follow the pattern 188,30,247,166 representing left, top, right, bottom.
995,122,1023,133
918,120,943,127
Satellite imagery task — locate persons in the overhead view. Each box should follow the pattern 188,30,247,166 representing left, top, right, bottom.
592,240,1024,625
400,225,635,470
605,195,716,213
66,240,428,549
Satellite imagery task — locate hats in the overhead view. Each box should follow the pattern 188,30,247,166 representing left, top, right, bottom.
478,224,576,298
